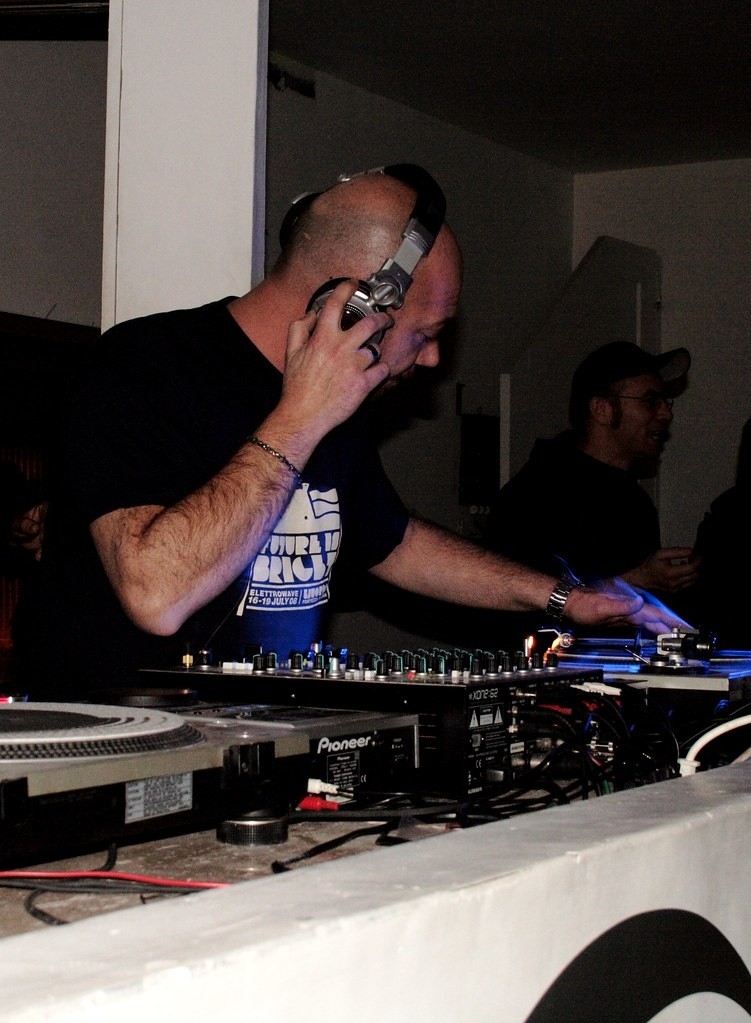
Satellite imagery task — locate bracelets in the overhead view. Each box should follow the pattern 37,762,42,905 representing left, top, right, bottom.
245,435,302,490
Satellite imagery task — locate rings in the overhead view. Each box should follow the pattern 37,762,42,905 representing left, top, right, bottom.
361,345,379,364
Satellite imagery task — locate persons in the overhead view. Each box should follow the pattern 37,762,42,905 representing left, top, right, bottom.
11,176,694,868
492,343,691,656
691,419,751,650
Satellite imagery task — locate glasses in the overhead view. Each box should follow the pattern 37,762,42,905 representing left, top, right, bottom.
602,389,673,412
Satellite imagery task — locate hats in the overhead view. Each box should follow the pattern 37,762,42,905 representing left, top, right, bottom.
567,341,692,422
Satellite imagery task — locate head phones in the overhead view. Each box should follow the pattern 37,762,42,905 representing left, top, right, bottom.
274,160,446,347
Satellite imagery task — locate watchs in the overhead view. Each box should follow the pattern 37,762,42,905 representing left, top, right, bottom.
546,574,586,623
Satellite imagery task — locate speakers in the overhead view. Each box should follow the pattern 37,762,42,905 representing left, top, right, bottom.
458,411,499,507
